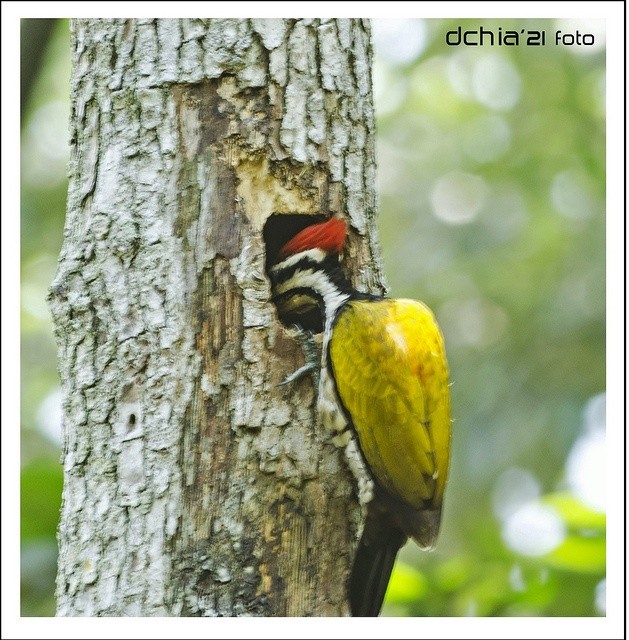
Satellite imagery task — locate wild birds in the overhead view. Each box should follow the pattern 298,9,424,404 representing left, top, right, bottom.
266,213,458,618
